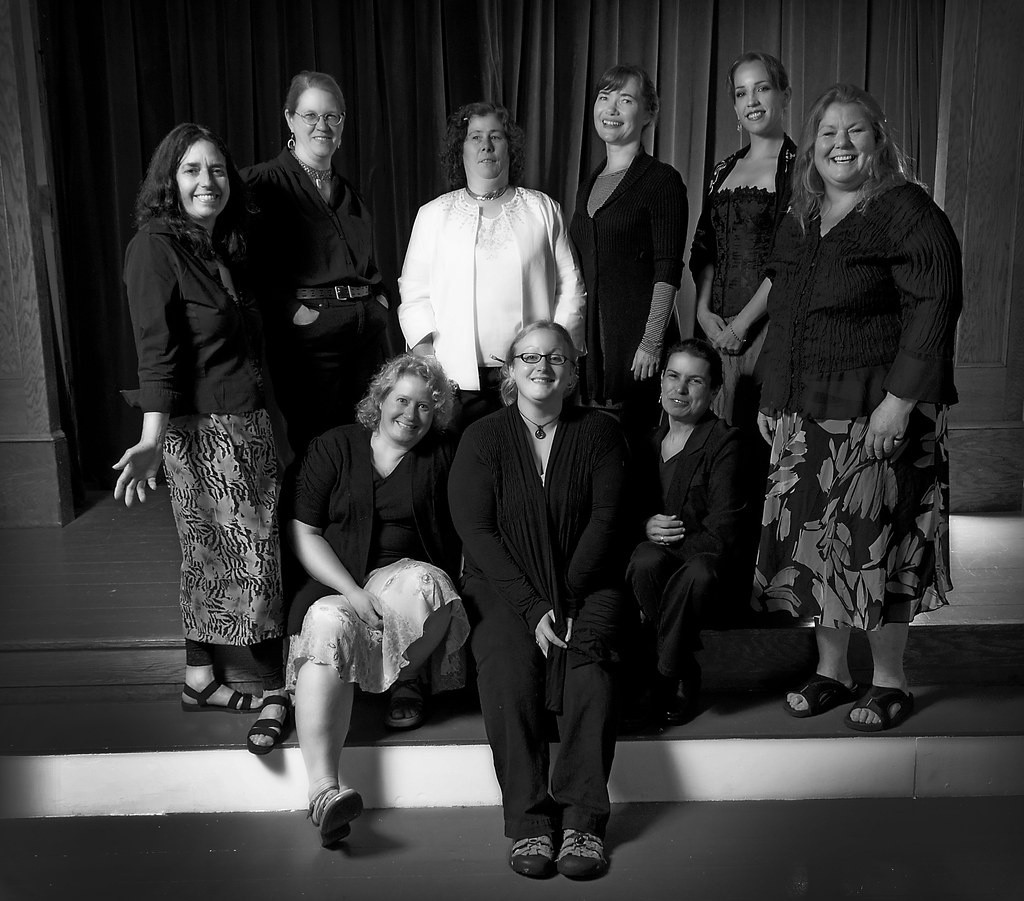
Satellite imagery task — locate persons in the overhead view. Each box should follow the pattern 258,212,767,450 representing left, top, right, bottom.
111,125,295,755
621,339,764,729
447,320,636,881
237,52,802,630
284,355,471,846
750,83,965,730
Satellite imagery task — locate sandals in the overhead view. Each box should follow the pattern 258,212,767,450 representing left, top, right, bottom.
247,696,288,755
181,677,263,714
388,678,424,729
306,784,363,847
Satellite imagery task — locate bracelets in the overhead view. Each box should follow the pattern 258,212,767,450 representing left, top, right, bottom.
729,322,746,342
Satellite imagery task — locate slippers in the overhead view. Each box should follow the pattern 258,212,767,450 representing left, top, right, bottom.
844,686,914,732
782,674,856,719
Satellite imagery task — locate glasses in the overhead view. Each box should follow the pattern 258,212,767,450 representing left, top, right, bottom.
514,352,576,365
295,110,344,126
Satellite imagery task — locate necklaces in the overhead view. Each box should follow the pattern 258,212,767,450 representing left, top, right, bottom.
519,410,560,439
465,185,511,201
291,149,333,189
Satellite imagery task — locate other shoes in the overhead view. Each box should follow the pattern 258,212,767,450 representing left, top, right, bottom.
662,679,700,725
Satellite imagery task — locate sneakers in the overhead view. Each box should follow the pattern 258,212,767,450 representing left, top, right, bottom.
556,829,610,878
509,835,554,876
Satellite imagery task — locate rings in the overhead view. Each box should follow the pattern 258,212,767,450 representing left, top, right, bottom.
894,437,904,441
661,535,664,541
536,637,539,644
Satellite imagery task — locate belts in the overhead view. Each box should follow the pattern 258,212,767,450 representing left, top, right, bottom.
297,284,370,301
